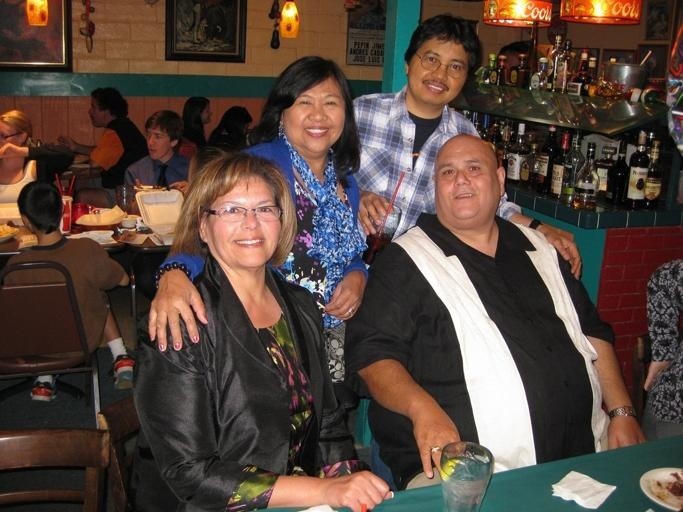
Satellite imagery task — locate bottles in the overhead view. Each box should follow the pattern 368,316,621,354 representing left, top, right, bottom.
484,34,601,98
459,108,664,211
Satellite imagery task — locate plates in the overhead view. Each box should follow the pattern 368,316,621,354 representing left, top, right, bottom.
133,185,167,192
0,228,19,244
72,153,89,163
639,466,683,512
74,231,122,245
75,213,124,227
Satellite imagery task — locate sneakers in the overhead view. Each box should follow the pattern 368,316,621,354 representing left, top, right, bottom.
30,380,57,402
112,355,136,389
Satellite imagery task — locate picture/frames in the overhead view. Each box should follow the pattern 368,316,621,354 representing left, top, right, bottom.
163,0,248,64
1,0,74,74
569,0,683,80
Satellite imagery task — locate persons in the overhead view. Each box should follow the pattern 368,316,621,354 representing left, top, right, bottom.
147,56,369,409
640,259,683,441
1,95,253,203
342,133,649,491
134,154,394,512
347,14,582,280
1,181,134,402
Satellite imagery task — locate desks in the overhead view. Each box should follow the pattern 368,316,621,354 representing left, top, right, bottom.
259,420,682,512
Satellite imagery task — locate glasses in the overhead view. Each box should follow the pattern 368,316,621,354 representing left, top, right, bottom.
418,55,467,79
213,206,283,222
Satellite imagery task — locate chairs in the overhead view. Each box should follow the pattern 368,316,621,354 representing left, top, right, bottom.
93,397,140,512
0,428,112,511
1,257,105,417
122,242,174,379
74,185,111,211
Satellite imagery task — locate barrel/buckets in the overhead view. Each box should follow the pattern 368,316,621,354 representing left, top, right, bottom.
603,62,649,86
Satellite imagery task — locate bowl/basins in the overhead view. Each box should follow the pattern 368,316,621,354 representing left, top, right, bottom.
121,214,139,228
604,62,649,89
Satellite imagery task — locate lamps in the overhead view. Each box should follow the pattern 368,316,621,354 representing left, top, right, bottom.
268,0,299,50
481,0,553,29
558,0,644,27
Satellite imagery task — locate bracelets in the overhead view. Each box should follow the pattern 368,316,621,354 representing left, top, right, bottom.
529,219,543,230
154,261,191,289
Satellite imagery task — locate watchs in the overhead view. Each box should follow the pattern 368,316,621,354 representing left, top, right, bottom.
609,406,637,421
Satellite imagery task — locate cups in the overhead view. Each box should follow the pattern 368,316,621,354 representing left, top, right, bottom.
58,195,73,234
439,441,494,511
115,185,131,212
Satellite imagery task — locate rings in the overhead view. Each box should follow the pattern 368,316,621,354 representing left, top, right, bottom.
431,445,442,452
349,308,354,314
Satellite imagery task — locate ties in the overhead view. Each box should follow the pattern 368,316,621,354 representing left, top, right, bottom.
157,163,167,191
